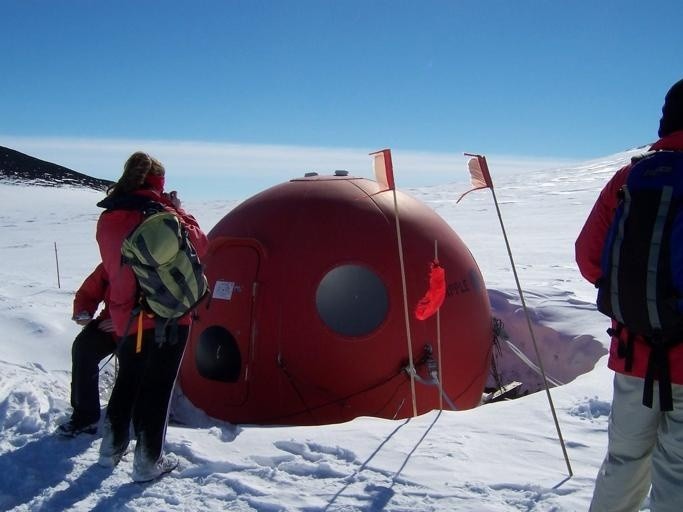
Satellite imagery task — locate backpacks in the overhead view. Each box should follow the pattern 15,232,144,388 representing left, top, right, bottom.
116,202,209,355
596,150,681,412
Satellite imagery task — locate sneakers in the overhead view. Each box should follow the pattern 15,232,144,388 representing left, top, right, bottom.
96,448,124,469
131,452,178,482
57,417,100,436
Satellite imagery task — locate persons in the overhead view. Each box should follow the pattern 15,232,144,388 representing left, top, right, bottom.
55,261,122,438
572,78,682,511
94,150,210,484
